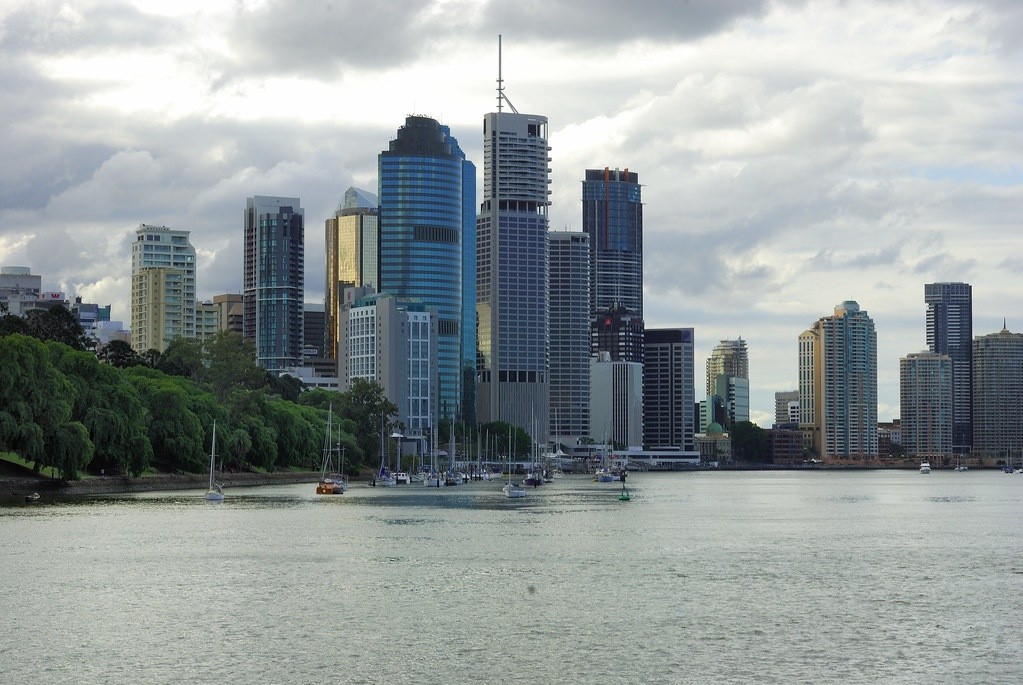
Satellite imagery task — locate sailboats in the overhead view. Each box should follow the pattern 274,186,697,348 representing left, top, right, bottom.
368,409,501,488
502,398,569,498
205,419,224,499
316,402,348,495
592,430,628,483
1001,440,1023,473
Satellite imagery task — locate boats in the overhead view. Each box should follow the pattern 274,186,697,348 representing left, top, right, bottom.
25,492,40,501
953,453,969,472
919,460,931,474
